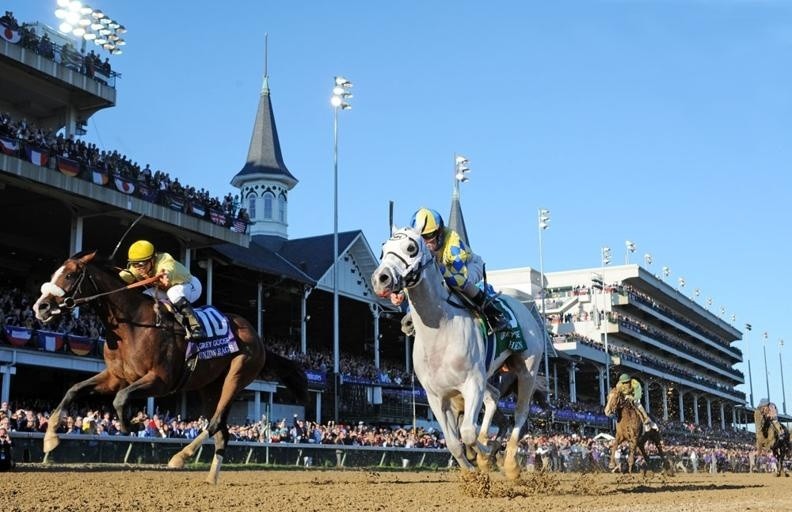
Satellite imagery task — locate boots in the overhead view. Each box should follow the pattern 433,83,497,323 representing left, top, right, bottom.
638,404,651,425
471,288,507,336
173,296,207,341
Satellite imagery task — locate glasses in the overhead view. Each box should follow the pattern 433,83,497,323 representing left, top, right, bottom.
421,231,440,240
131,262,145,269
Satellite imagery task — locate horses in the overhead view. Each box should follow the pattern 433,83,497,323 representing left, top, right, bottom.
369,224,545,486
30,249,312,485
752,402,792,477
602,386,670,472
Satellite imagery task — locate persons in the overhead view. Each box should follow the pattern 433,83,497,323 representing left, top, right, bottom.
373,361,614,473
389,204,512,337
535,279,746,400
757,398,786,438
1,279,374,473
1,8,112,86
615,414,792,473
616,374,652,425
0,110,255,233
117,238,206,341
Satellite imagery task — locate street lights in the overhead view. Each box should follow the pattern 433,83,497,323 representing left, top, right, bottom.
328,75,352,376
600,247,612,391
536,207,553,405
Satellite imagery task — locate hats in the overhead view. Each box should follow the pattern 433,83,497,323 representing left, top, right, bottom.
759,398,770,406
619,373,631,383
409,207,444,235
127,240,156,262
